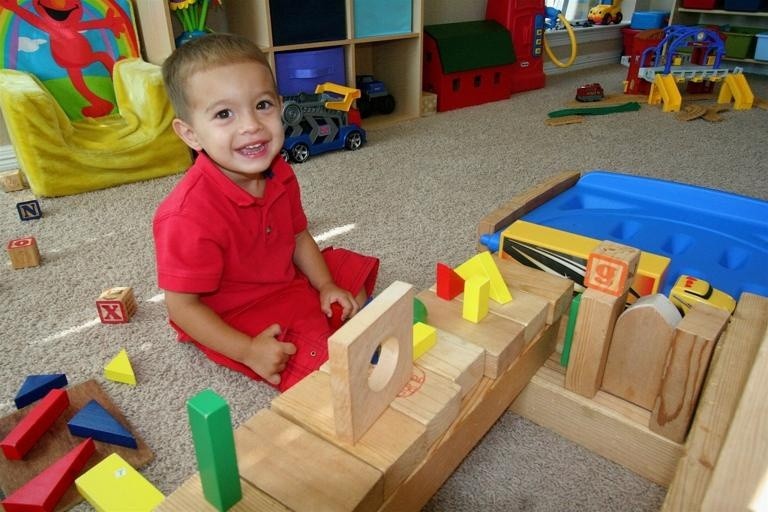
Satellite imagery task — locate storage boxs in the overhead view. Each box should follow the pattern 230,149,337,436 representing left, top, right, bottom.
671,0,767,61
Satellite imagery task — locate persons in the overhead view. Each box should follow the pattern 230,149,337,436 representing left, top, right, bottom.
153,33,379,393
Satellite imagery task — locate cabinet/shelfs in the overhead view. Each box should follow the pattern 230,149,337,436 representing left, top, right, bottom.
135,0,425,133
661,0,767,70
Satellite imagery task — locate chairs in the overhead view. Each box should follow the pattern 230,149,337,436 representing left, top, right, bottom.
0,0,195,198
